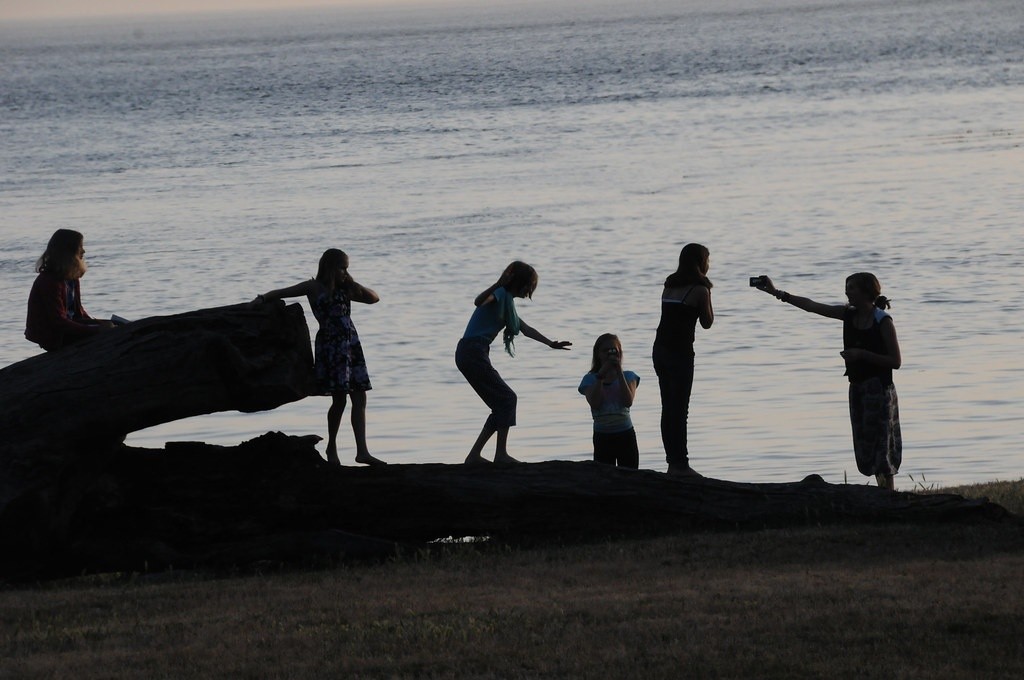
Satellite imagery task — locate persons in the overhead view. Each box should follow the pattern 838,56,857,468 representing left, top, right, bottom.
249,248,387,466
23,229,115,352
578,334,641,469
757,272,902,489
653,243,715,478
456,262,573,464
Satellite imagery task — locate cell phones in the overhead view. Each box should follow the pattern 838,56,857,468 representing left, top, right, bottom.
750,277,765,287
608,348,617,358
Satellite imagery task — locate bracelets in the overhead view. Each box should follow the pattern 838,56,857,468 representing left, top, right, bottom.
596,375,605,379
769,288,793,303
